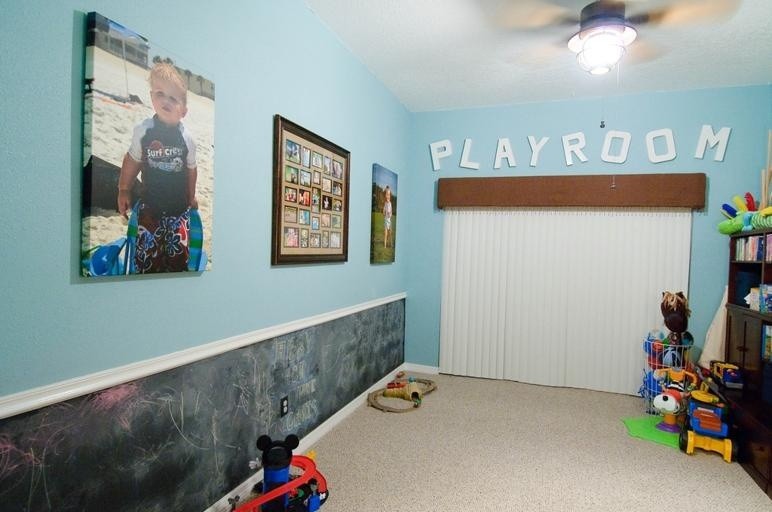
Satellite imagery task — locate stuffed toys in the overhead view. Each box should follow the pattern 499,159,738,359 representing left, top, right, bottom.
717,192,772,235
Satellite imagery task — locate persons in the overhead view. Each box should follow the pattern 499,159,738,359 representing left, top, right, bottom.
118,63,198,274
383,186,392,248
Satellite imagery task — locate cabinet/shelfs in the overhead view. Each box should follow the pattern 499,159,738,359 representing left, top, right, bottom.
696,232,771,500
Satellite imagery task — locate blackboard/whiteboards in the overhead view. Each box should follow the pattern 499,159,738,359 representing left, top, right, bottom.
0,291,407,512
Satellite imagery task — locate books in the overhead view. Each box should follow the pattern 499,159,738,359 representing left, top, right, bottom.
735,234,772,262
762,324,772,360
750,284,772,312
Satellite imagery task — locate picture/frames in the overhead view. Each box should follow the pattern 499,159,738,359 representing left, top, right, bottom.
271,114,350,264
